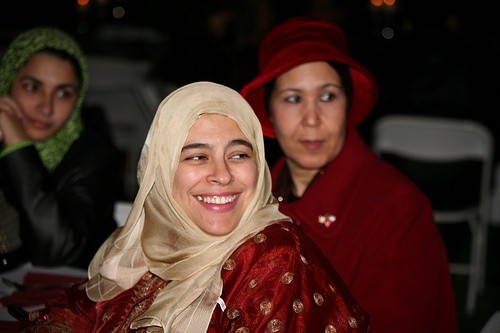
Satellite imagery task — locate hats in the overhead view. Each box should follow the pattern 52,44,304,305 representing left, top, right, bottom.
85,81,291,333
239,18,378,139
0,29,86,173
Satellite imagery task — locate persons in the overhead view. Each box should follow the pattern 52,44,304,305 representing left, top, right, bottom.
20,80,363,333
0,28,119,271
240,21,456,333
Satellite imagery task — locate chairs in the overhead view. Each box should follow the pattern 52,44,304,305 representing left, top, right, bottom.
372,116,491,314
88,58,159,175
